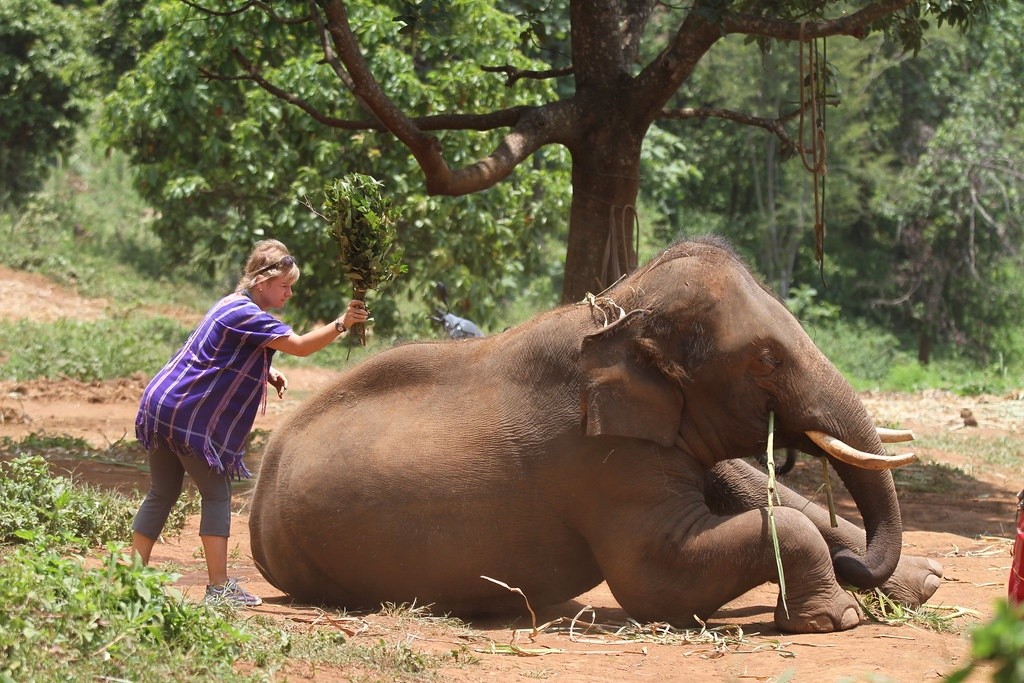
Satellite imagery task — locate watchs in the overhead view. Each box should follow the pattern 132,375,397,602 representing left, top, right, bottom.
335,318,347,332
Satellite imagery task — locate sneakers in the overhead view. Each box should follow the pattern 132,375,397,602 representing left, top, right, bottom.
204,578,262,606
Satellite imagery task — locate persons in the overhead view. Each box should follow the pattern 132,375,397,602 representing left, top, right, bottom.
129,238,369,606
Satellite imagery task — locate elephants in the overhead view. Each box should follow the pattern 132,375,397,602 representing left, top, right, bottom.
248,231,944,635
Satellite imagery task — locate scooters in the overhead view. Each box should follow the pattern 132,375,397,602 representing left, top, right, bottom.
425,282,510,340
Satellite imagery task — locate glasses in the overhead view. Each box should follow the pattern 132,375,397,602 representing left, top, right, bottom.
251,256,296,279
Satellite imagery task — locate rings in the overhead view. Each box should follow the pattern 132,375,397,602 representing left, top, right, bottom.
354,313,357,318
